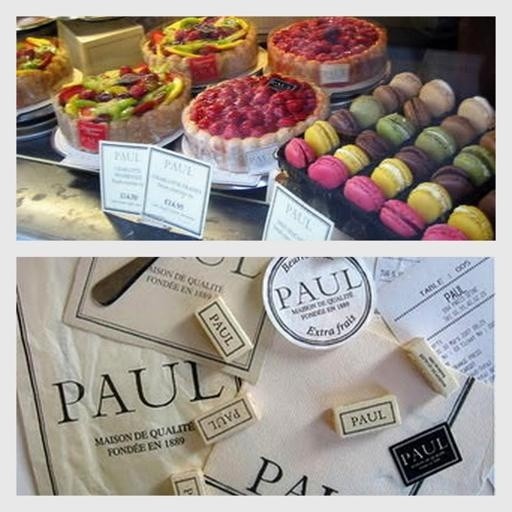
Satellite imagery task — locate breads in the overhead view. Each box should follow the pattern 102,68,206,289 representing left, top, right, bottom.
457,95,495,135
402,96,432,131
371,157,414,199
333,143,371,176
354,128,393,161
453,144,495,188
389,71,422,98
376,112,415,147
447,204,494,241
328,108,359,137
342,174,385,214
304,119,340,157
349,95,387,130
421,223,467,240
284,137,316,170
440,114,477,149
406,181,453,225
394,145,434,178
419,78,456,118
478,189,495,226
307,154,349,191
414,126,457,166
372,84,406,114
479,130,495,151
429,164,475,202
379,199,425,239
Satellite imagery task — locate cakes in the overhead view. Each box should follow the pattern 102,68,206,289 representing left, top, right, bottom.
52,63,192,155
17,34,75,109
266,17,389,95
140,17,259,85
180,73,331,175
